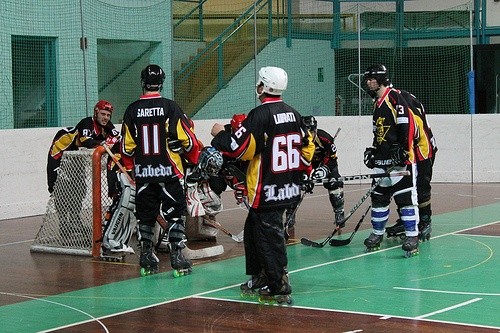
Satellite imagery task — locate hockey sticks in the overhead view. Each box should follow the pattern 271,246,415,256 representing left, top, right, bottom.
300,170,411,185
330,202,371,246
285,128,341,241
102,140,224,259
201,214,244,244
300,166,394,248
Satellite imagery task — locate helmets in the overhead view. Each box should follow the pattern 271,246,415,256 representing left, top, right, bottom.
141,64,165,89
230,113,248,132
197,146,223,180
94,100,113,121
363,65,390,84
301,116,317,131
256,66,288,95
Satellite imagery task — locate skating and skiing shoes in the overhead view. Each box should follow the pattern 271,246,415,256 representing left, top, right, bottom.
139,241,160,276
288,226,295,243
258,266,293,305
334,209,345,234
386,219,406,239
402,237,420,258
240,270,267,299
364,233,383,253
417,220,431,242
170,249,193,277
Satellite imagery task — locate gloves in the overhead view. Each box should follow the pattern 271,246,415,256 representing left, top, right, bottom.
364,147,376,169
312,165,330,180
300,174,311,191
389,143,411,172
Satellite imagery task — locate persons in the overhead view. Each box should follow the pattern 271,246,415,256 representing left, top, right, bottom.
102,142,223,255
210,66,315,307
364,64,420,258
211,113,250,204
385,79,438,242
120,65,201,277
284,116,346,234
47,101,120,238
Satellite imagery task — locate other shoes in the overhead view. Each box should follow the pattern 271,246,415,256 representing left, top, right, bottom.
70,221,92,233
59,227,84,238
